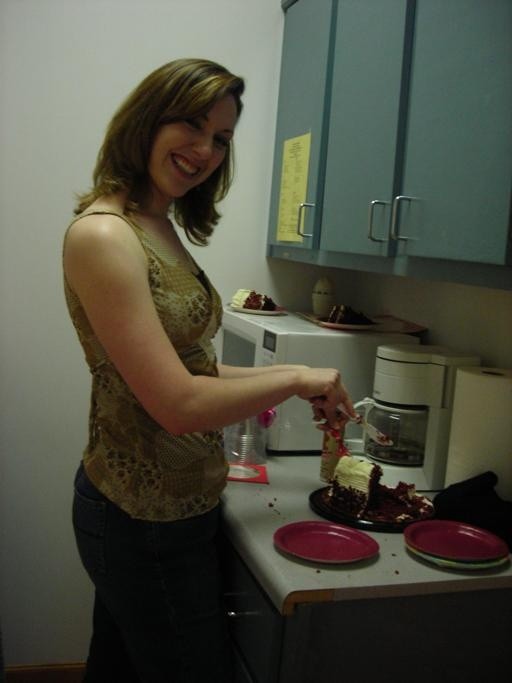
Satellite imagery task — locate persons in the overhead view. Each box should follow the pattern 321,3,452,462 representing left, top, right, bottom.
60,56,360,682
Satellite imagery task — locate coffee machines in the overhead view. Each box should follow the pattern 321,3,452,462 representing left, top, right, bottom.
352,344,480,498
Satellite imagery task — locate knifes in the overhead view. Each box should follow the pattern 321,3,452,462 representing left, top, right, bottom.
336,403,394,447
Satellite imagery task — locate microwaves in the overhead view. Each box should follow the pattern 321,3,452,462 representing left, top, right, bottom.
212,304,420,453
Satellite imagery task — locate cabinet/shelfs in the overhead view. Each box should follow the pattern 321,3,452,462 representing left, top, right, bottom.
267,1,512,293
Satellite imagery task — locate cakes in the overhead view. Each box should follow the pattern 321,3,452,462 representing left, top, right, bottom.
232,288,280,310
328,456,383,520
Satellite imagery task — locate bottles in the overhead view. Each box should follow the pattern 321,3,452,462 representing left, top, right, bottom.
319,415,347,484
312,276,342,316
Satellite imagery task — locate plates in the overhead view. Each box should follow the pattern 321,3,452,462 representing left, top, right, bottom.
229,303,283,315
316,315,381,330
403,520,511,572
272,520,380,563
307,484,436,531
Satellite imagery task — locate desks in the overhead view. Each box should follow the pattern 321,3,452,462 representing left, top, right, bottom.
200,449,511,679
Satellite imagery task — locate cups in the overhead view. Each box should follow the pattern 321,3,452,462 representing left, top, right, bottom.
223,413,261,466
